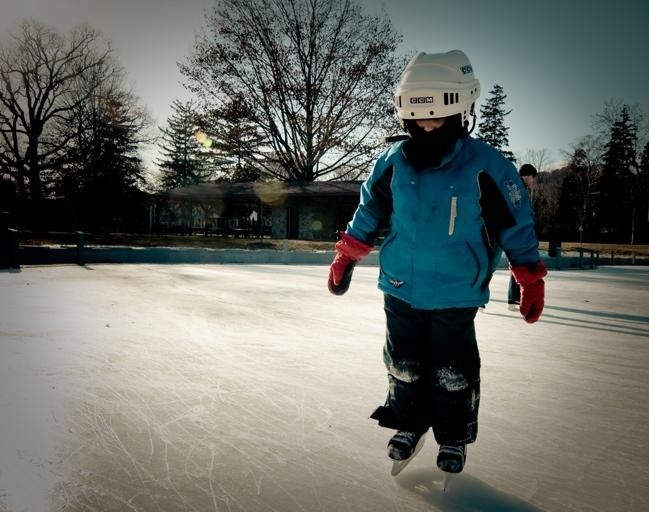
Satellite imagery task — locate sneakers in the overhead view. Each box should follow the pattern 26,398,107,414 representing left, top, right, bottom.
436,445,468,473
386,430,421,460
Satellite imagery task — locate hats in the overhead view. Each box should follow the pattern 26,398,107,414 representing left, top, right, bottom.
519,164,537,176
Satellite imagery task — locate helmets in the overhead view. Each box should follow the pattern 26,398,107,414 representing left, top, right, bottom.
392,49,481,133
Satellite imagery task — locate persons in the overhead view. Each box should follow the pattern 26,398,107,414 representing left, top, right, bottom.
325,50,549,476
506,162,538,307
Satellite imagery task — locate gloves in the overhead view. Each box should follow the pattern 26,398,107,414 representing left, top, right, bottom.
507,260,548,324
327,231,373,295
